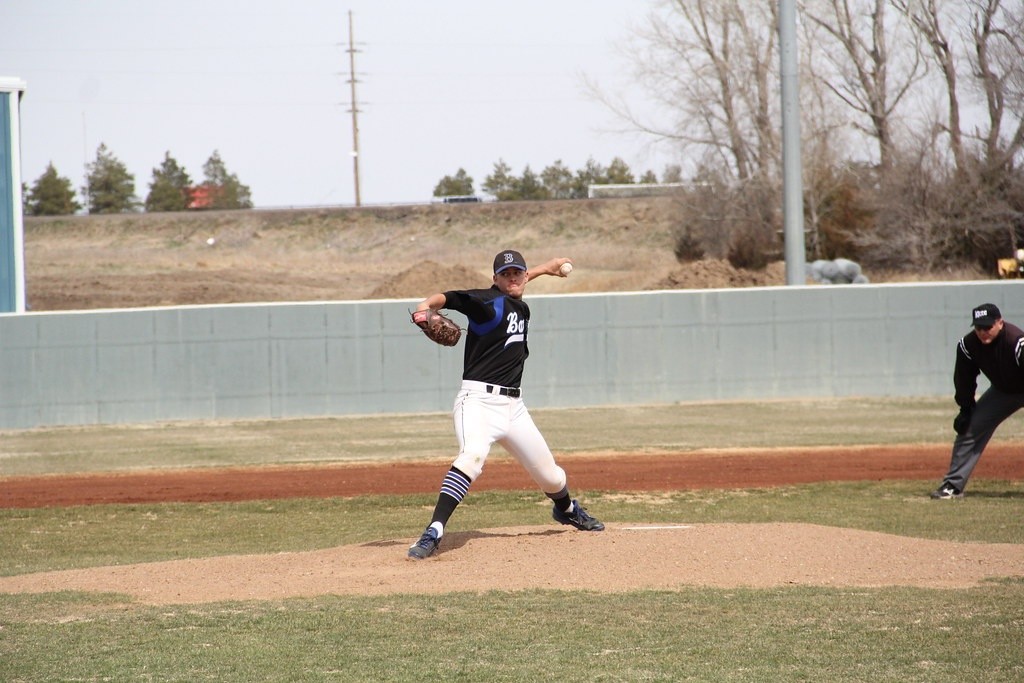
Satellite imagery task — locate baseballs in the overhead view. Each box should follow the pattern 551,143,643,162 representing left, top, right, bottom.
560,263,573,275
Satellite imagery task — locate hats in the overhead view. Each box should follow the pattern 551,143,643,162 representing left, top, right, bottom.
493,249,527,276
971,304,1001,327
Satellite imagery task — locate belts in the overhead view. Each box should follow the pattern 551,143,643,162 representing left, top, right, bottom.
486,385,520,397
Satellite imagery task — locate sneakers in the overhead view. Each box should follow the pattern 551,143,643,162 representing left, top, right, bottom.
929,481,963,500
408,527,440,559
555,499,605,532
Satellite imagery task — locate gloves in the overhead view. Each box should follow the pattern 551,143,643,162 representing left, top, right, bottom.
953,407,975,435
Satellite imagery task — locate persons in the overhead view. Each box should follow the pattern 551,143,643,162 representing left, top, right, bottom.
409,249,605,558
930,303,1024,499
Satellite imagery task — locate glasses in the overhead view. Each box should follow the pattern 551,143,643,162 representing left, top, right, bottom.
975,319,998,331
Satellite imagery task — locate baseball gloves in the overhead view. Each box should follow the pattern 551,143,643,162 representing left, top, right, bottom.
407,306,467,348
